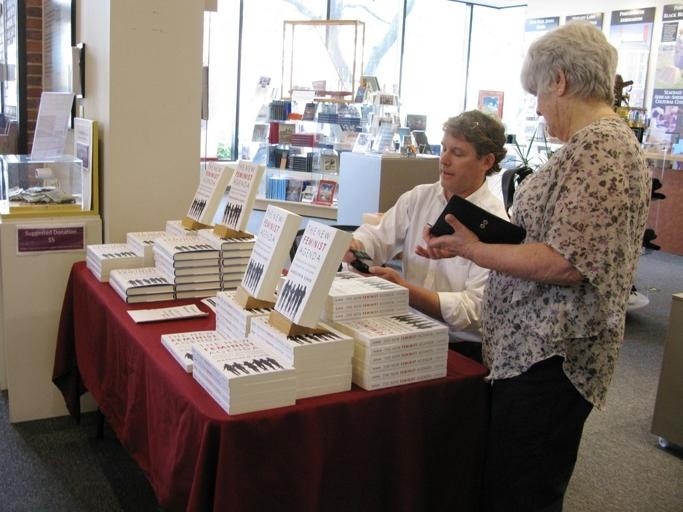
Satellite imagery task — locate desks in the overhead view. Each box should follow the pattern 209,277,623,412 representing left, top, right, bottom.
52,261,489,512
652,294,683,452
0,216,102,424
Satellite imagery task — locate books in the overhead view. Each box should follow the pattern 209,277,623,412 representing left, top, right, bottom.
425,194,526,258
246,65,436,215
87,160,450,417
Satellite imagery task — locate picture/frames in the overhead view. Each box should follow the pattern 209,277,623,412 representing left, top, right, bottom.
478,90,503,118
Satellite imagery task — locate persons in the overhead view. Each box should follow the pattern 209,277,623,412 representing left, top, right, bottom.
339,110,517,353
415,21,652,512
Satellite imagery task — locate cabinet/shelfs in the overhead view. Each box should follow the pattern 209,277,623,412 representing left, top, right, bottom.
252,119,352,220
